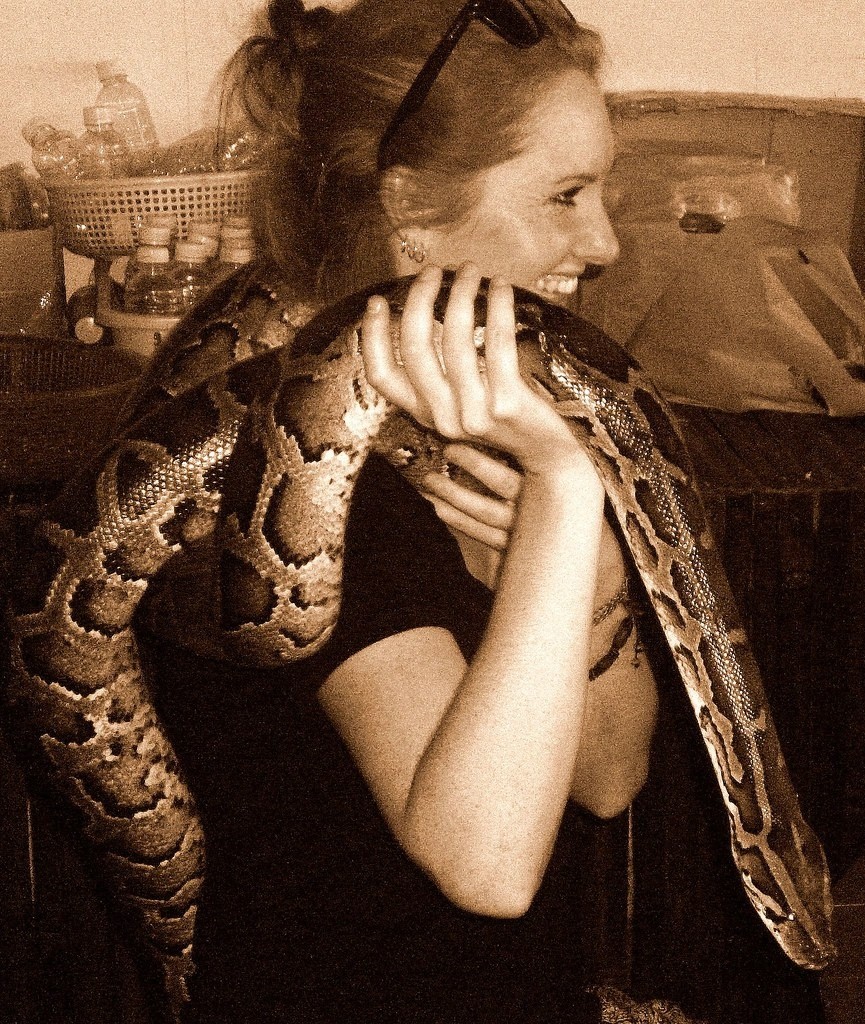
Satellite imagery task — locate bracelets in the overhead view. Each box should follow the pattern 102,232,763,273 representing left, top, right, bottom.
589,572,649,681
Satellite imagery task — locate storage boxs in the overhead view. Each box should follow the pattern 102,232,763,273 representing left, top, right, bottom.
0,219,68,339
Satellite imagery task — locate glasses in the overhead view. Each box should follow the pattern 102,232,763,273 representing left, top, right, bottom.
376,0,575,169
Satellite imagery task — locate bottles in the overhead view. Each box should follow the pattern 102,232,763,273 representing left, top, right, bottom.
21,58,260,346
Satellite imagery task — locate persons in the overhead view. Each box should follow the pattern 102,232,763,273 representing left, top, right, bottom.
206,2,657,1024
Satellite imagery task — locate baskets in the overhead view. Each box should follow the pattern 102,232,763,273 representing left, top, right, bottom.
41,168,270,261
0,331,148,488
